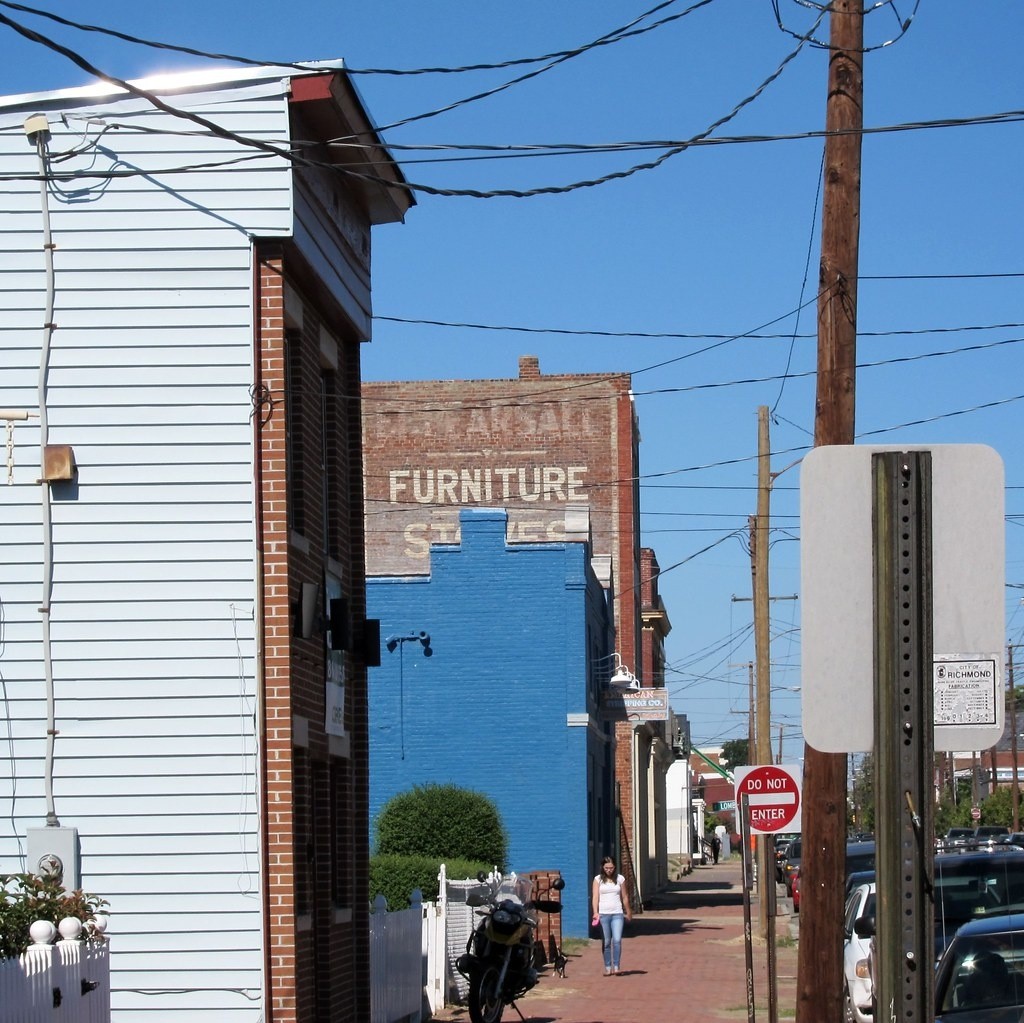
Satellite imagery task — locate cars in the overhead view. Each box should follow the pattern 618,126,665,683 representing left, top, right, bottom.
929,910,1024,1023
773,833,1024,1023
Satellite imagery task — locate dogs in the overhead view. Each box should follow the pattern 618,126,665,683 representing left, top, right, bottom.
552,955,568,978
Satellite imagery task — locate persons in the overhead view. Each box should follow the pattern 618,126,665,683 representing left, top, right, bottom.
711,834,721,865
592,856,632,975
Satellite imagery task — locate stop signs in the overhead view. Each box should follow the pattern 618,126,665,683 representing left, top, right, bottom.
732,763,802,838
971,808,980,819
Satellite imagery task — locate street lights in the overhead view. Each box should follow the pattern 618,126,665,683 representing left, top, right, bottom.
749,687,800,887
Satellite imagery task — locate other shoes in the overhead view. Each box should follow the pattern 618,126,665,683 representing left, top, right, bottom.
603,970,622,976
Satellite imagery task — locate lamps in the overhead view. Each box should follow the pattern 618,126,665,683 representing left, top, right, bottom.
589,653,641,695
385,630,430,645
293,581,320,640
320,598,350,650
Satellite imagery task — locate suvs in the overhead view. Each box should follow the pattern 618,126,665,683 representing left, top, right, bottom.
944,829,973,852
968,827,1009,850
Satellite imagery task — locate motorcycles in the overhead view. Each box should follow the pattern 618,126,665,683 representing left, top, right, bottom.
455,871,565,1023
